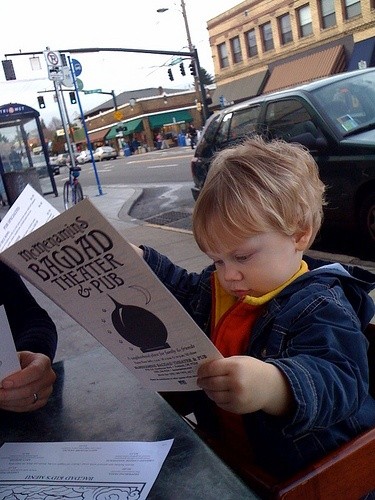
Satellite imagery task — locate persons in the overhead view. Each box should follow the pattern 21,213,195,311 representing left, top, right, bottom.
0,137,375,500
121,125,197,152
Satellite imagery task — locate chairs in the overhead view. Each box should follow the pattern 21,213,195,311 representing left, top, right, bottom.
158,323,375,500
292,122,326,143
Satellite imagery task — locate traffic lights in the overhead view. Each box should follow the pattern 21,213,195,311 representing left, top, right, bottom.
37,96,46,108
189,62,196,76
123,126,127,131
69,92,77,104
167,68,174,81
179,62,186,77
116,127,120,132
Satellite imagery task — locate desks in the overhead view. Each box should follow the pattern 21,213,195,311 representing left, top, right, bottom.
0,345,255,500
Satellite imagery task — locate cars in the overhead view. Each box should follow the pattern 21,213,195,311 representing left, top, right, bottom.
189,68,375,249
92,146,117,162
66,153,81,165
22,155,60,177
76,150,94,164
58,154,68,166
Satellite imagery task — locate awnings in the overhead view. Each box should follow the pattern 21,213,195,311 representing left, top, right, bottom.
82,109,193,143
208,36,375,110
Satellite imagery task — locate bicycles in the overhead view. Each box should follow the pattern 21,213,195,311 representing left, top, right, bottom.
62,161,83,211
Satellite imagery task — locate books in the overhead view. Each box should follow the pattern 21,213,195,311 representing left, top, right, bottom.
0,184,225,393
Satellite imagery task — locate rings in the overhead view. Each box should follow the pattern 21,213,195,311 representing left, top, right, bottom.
33,393,38,404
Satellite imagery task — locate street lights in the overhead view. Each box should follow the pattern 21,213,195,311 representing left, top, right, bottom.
157,0,209,121
